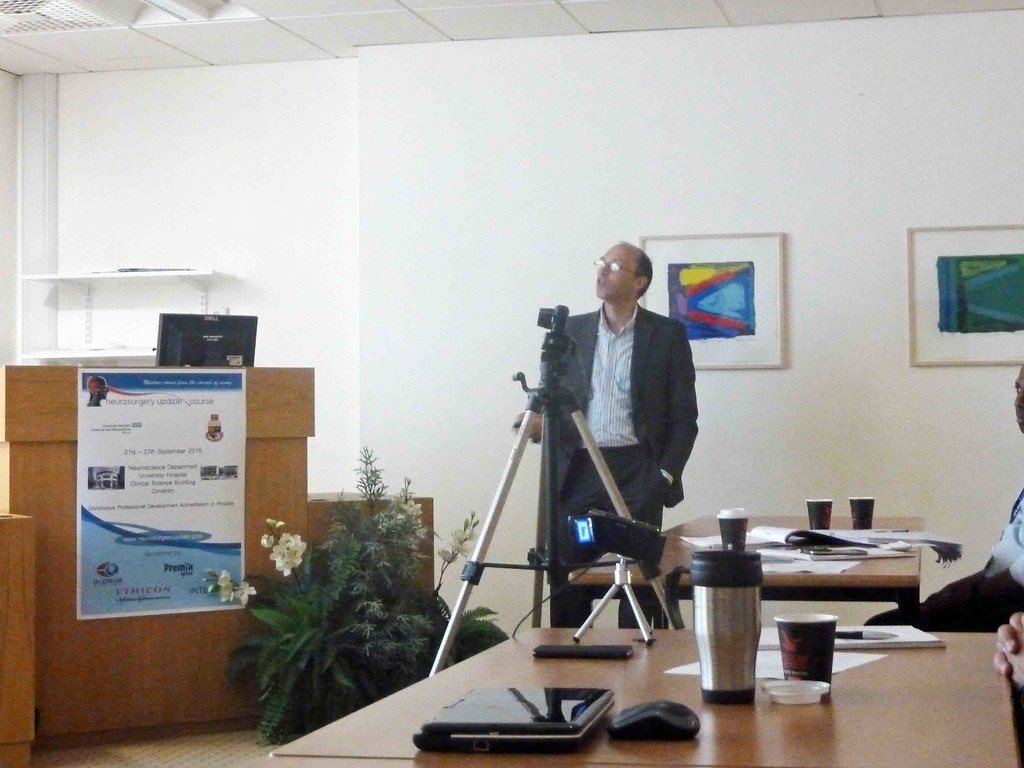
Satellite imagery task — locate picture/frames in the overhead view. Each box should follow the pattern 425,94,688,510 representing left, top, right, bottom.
907,225,1024,368
639,232,789,370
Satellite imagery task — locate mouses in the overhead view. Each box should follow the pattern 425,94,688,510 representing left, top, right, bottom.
608,699,701,740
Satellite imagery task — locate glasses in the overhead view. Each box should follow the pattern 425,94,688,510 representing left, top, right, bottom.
594,257,648,277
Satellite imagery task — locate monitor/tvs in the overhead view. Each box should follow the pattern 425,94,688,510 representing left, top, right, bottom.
156,313,258,366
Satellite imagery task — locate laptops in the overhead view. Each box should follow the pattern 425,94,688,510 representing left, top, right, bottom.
413,686,614,752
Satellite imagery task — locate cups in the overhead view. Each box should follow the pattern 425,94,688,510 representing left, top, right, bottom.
773,613,839,703
806,499,833,530
850,496,875,530
717,508,750,551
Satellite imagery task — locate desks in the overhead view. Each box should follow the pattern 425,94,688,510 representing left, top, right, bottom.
569,514,923,631
268,626,1023,768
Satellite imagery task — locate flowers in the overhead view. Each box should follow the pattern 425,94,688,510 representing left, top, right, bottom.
196,452,505,745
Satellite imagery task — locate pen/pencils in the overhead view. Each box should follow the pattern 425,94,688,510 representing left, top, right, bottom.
835,630,900,640
874,529,909,533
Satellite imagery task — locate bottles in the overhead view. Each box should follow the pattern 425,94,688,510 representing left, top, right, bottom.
208,414,221,438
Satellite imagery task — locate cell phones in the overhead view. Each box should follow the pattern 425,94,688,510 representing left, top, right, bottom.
533,645,634,660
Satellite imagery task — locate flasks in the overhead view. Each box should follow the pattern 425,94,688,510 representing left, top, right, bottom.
691,551,763,704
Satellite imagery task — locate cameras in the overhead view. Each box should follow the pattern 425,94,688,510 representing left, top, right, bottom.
537,304,569,332
566,507,666,565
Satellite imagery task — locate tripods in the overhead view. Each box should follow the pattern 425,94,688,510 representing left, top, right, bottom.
427,331,675,680
572,555,655,645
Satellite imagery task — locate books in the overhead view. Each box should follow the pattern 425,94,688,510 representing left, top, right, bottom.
681,525,962,570
758,625,946,651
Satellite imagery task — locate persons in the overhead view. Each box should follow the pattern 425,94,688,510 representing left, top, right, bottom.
514,240,698,628
864,364,1024,633
993,610,1024,709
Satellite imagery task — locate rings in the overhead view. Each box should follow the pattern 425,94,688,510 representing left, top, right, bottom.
1003,647,1006,654
